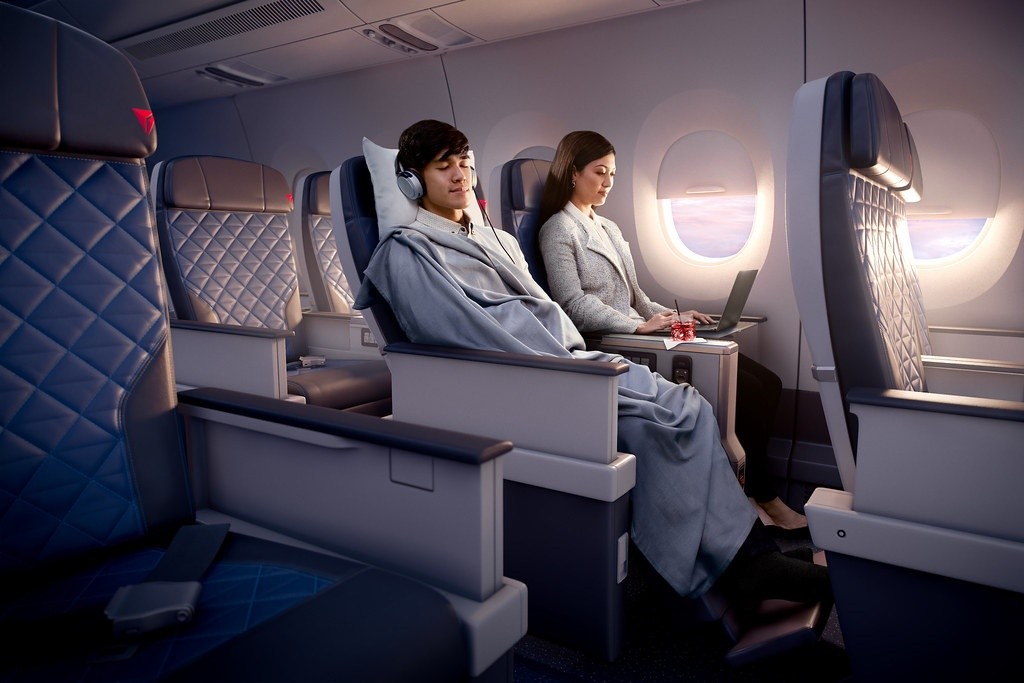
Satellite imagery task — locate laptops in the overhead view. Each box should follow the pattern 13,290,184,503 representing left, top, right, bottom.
655,269,759,332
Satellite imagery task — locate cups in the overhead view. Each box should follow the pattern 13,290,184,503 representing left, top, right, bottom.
671,313,697,342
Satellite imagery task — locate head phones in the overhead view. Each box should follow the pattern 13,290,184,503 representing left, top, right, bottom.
395,148,477,200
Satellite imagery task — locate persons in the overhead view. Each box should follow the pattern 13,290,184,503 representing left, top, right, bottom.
355,119,830,632
532,130,812,545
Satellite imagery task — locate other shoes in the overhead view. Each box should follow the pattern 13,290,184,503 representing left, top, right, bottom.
744,551,832,613
765,523,812,541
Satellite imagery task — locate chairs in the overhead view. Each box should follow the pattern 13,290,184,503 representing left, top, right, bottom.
2,2,1024,682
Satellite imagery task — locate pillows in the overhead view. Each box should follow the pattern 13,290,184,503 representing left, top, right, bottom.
359,136,484,240
